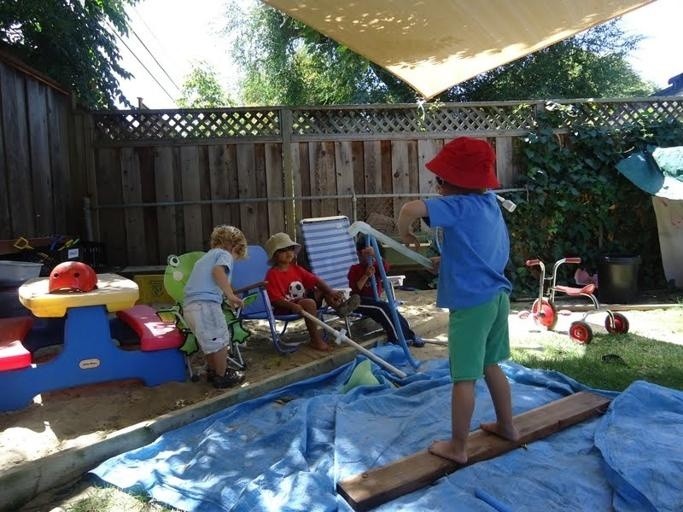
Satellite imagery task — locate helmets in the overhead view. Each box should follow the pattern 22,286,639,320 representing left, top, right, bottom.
48,261,98,294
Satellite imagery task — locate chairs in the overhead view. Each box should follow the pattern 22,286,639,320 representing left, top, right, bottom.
229,245,349,353
299,215,406,346
155,251,258,382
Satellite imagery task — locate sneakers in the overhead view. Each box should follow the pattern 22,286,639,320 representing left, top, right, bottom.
206,365,231,380
337,294,361,317
212,369,243,389
412,336,426,347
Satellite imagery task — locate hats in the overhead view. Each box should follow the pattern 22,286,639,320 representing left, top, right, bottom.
264,232,301,264
426,136,498,192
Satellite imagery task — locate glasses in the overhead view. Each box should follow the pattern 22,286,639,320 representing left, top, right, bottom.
434,175,450,187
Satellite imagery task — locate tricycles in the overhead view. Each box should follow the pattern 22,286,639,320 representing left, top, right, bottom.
519,256,629,344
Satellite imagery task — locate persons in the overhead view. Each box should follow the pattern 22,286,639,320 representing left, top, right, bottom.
346,232,426,348
262,230,361,352
181,223,248,387
396,133,521,465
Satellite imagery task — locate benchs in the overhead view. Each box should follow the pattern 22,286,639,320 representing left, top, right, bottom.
116,303,187,353
0,315,34,373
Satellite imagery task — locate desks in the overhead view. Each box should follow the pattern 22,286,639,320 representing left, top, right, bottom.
0,273,186,410
